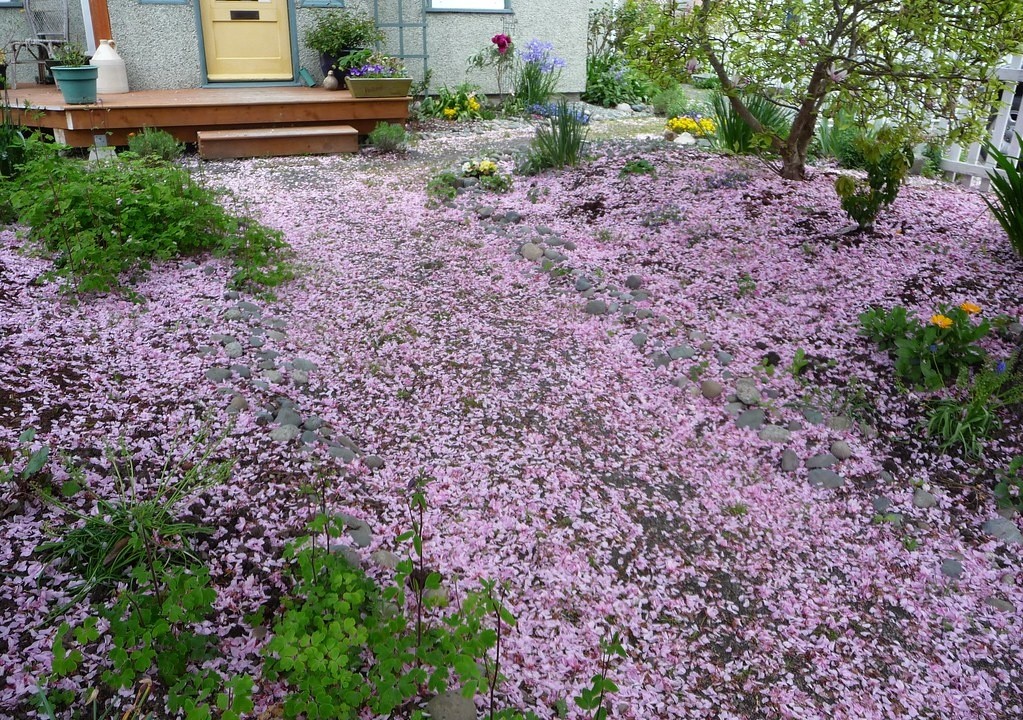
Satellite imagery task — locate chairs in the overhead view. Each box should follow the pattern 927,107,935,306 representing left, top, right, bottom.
9,0,69,90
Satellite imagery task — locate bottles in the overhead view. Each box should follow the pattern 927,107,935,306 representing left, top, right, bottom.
323,70,338,90
89,39,129,94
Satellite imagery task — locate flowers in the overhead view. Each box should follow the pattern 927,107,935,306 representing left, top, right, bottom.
346,54,408,78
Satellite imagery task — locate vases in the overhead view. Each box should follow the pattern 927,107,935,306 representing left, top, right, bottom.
344,74,413,99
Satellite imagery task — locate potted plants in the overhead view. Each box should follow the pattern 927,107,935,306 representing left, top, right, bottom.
302,0,389,87
0,49,9,90
45,36,98,105
